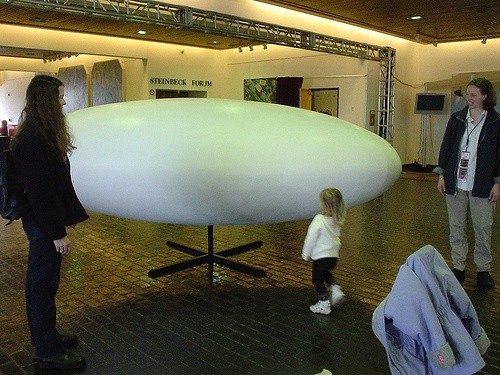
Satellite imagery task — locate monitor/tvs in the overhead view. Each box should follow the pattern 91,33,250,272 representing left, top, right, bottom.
414,92,448,115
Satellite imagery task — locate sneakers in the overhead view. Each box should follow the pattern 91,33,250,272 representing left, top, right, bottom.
476,270,495,287
452,268,466,282
331,283,346,306
309,299,332,315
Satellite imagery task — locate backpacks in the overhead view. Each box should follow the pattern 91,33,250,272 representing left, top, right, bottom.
0,137,32,226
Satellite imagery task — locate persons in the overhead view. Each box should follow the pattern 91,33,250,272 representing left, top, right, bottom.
301,188,346,314
10,74,90,369
0,120,8,136
433,77,500,287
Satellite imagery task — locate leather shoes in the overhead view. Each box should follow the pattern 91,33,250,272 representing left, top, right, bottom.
31,331,78,351
34,350,86,370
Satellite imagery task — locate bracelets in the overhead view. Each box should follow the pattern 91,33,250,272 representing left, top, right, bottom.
439,177,444,180
